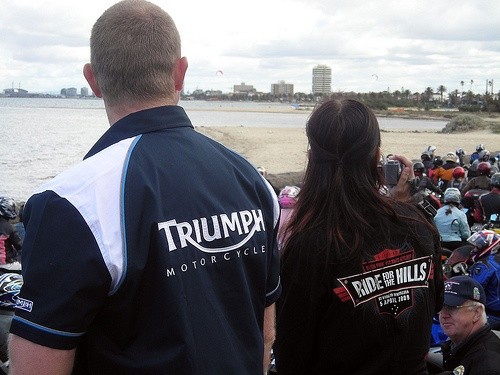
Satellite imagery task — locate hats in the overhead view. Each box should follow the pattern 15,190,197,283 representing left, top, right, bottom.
443,274,486,308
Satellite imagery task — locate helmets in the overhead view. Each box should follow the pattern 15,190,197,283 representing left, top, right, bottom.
456,148,465,156
413,162,424,176
478,150,490,162
444,187,461,203
421,151,432,161
490,173,500,186
0,196,17,219
476,144,484,152
447,152,457,162
477,162,491,176
278,185,301,209
471,230,500,263
425,145,437,152
452,167,465,179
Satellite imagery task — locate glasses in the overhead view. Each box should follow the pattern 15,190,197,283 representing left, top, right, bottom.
437,304,477,315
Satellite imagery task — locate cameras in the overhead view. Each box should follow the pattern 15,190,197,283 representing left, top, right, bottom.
376,159,399,185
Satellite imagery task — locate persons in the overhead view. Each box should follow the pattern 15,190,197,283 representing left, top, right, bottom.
278,186,301,249
426,230,500,375
410,145,500,266
0,195,25,375
9,0,281,375
273,97,444,375
